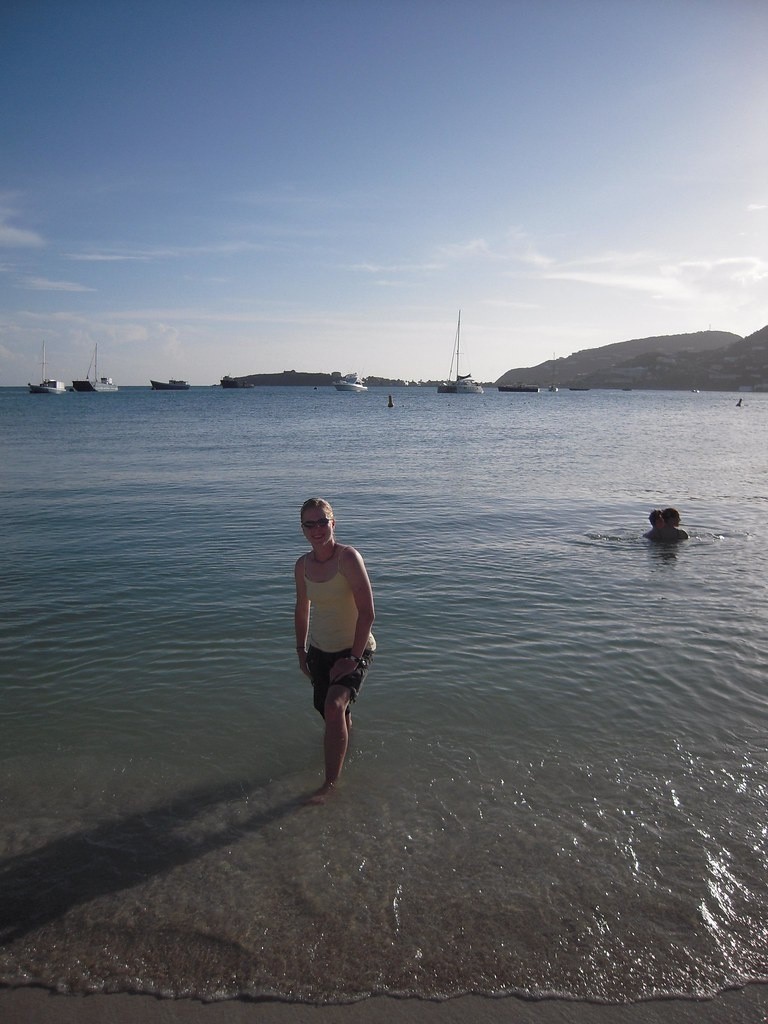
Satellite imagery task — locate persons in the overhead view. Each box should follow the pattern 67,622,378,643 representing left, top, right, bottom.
294,498,378,806
643,508,688,542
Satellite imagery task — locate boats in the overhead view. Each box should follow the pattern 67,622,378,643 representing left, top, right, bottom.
498,381,540,392
150,379,190,390
569,385,590,391
332,372,368,392
622,388,632,391
220,376,254,388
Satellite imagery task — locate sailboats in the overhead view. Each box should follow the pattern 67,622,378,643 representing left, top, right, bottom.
437,309,484,394
548,352,558,392
28,340,67,395
72,342,118,392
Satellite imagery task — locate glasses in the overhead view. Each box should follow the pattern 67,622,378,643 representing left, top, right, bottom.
302,518,335,528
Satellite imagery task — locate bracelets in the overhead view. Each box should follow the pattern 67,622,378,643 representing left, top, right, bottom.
348,653,361,662
296,647,305,649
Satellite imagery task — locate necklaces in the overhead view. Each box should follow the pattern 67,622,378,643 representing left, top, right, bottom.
314,543,336,563
